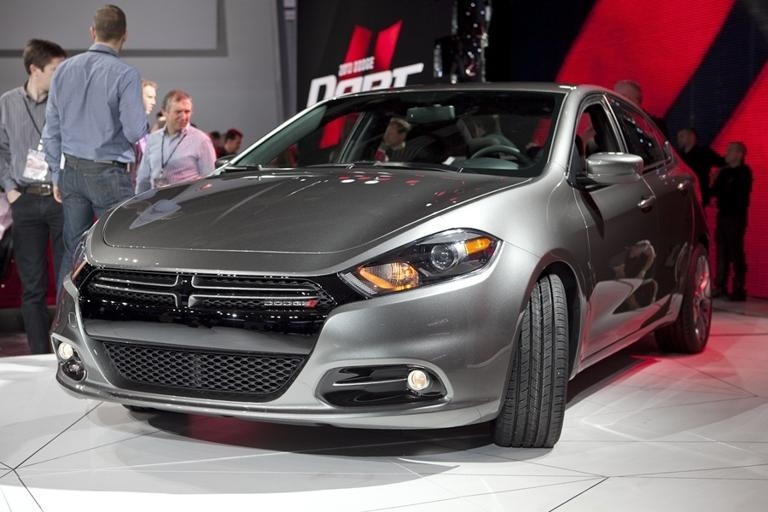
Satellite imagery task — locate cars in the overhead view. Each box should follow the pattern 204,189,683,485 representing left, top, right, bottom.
52,74,724,461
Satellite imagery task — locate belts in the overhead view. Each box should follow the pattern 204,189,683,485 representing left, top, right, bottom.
23,184,54,196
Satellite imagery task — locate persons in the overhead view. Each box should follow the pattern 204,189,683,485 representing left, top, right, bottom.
384,117,417,162
43,3,149,305
209,129,244,159
677,126,753,301
130,80,216,198
2,38,66,355
614,81,666,136
